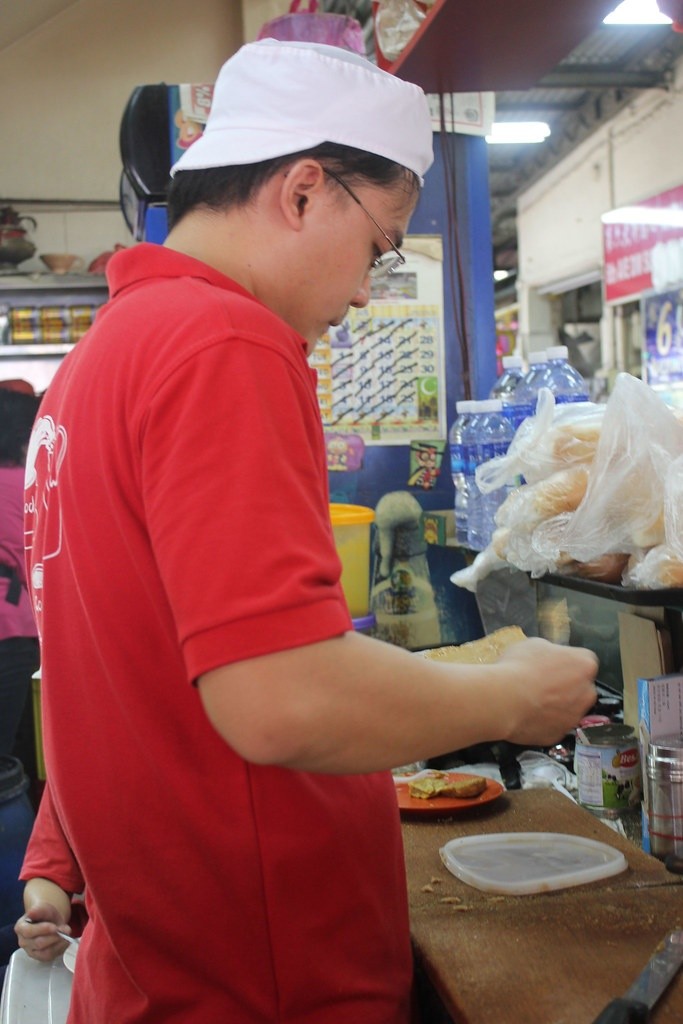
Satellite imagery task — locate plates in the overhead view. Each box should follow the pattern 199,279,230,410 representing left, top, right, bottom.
63,937,83,973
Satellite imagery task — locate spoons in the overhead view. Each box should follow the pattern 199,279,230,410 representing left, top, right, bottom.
534,766,578,805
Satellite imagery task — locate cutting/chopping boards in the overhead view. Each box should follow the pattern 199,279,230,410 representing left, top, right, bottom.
400,788,683,1024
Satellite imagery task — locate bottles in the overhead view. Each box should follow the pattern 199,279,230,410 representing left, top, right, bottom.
449,400,521,552
490,346,589,431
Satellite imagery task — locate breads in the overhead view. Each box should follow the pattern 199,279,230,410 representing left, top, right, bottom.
407,775,486,799
494,417,683,592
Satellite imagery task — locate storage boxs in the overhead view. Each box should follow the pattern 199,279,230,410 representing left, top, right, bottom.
324,503,375,623
421,510,457,546
462,545,683,724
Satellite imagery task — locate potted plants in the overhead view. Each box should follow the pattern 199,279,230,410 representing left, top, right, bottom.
0,208,36,273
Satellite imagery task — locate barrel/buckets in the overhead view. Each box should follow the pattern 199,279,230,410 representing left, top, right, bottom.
329,502,374,618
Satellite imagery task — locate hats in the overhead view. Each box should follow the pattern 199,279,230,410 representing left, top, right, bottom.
169,37,436,188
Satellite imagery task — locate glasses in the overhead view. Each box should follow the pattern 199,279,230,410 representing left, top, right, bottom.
284,166,407,278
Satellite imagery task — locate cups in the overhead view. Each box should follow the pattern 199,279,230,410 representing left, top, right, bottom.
353,616,374,636
40,254,83,273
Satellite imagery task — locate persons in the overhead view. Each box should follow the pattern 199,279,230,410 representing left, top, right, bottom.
26,38,599,1024
0,378,87,965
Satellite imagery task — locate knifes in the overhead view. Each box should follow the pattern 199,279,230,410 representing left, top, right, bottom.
592,928,683,1024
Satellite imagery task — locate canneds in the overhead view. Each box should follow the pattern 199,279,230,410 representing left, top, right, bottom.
575,724,642,818
